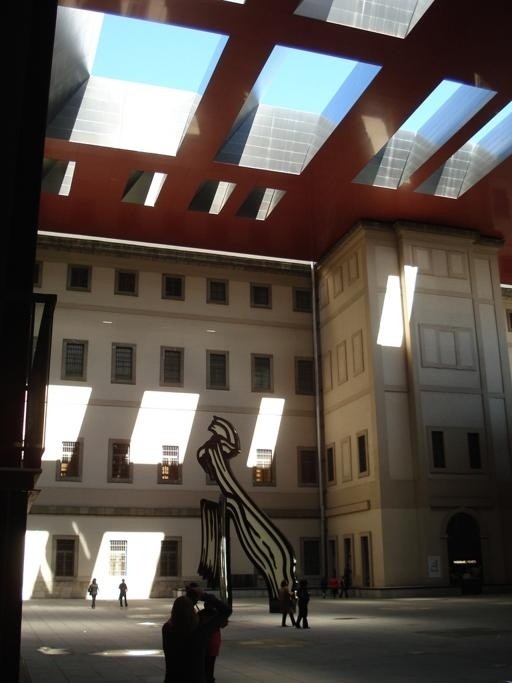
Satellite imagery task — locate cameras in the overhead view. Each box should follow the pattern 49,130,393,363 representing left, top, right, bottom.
187,584,200,603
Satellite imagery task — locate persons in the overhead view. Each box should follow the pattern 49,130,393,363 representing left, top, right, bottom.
277,579,295,627
161,584,232,683
117,578,130,607
201,596,221,683
89,578,99,609
330,574,340,596
295,578,310,630
339,576,349,598
